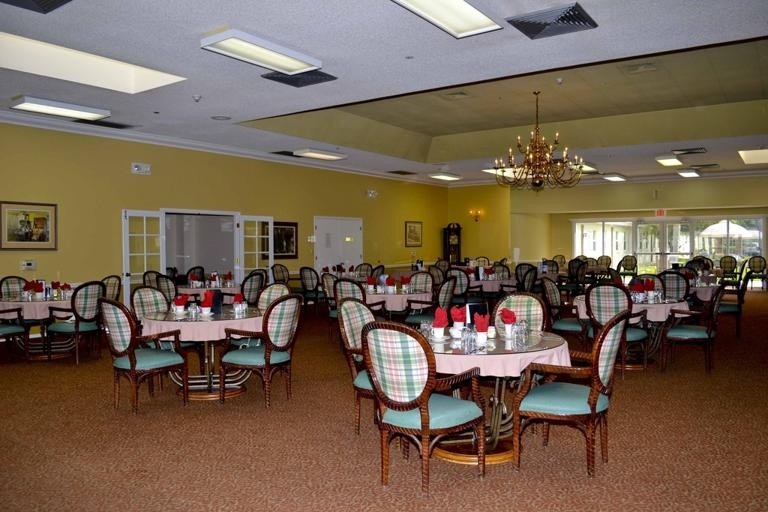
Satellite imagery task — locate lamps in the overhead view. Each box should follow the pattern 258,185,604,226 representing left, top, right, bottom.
427,172,463,182
293,148,349,161
393,0,504,39
200,29,322,76
654,155,703,178
8,97,111,121
600,173,627,182
493,90,584,191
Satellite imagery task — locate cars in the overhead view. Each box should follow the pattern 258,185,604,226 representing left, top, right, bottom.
745,242,760,256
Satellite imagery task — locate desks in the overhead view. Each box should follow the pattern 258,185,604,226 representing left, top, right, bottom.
136,305,265,402
402,329,573,465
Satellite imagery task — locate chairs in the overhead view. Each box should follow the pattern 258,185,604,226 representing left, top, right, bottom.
335,297,380,436
359,320,488,498
217,292,305,408
96,297,190,417
228,280,291,352
509,307,630,479
140,253,767,376
130,286,189,401
1,274,122,366
492,292,551,436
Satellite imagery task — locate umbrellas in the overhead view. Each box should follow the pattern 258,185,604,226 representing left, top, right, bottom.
698,219,752,254
734,230,762,240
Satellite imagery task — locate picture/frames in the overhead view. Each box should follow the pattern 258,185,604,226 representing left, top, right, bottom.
261,221,298,260
405,220,422,247
0,200,58,251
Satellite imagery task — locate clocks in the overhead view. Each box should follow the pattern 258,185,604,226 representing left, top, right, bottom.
442,222,463,264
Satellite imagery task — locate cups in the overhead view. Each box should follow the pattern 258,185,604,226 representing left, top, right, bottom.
188,278,236,288
20,280,74,301
361,281,412,296
320,270,358,280
626,289,664,304
169,301,249,319
420,320,531,352
